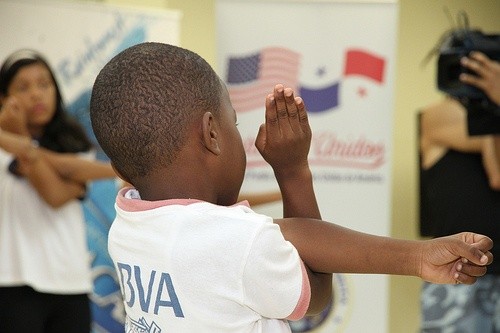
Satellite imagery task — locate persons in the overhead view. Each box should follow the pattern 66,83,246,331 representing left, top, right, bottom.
90,42,493,333
0,48,116,333
420,30,500,333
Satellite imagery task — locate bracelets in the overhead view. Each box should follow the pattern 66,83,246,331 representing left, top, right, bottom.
18,138,39,162
